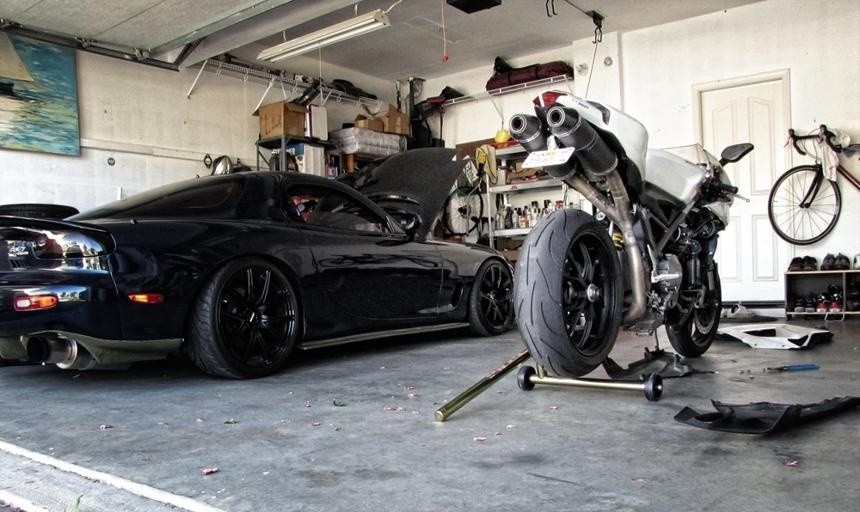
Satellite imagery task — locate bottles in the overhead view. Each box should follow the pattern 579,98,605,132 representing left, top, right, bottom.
495,199,575,229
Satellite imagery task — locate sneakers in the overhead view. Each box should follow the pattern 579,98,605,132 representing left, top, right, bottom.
853,254,860,270
720,303,754,319
821,254,834,271
804,297,816,312
816,295,828,312
833,254,850,270
846,281,860,312
793,296,805,312
803,256,816,271
788,257,803,271
828,294,842,312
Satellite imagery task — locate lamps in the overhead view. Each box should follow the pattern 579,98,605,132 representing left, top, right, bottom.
256,3,393,65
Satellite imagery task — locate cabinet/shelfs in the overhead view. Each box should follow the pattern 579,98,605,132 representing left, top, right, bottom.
256,133,343,180
485,146,582,248
343,152,376,172
783,269,860,321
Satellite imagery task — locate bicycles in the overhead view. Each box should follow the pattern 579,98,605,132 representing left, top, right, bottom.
766,121,860,248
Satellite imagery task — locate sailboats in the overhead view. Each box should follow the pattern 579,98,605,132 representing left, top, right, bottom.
0,30,35,97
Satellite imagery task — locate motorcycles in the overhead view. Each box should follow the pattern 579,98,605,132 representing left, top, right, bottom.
506,87,758,384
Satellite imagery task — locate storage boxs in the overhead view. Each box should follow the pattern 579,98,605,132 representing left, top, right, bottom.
252,100,410,140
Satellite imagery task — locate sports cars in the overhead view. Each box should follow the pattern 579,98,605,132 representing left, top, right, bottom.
2,138,518,386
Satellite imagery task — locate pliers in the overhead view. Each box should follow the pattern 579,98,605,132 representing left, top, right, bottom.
767,365,819,371
